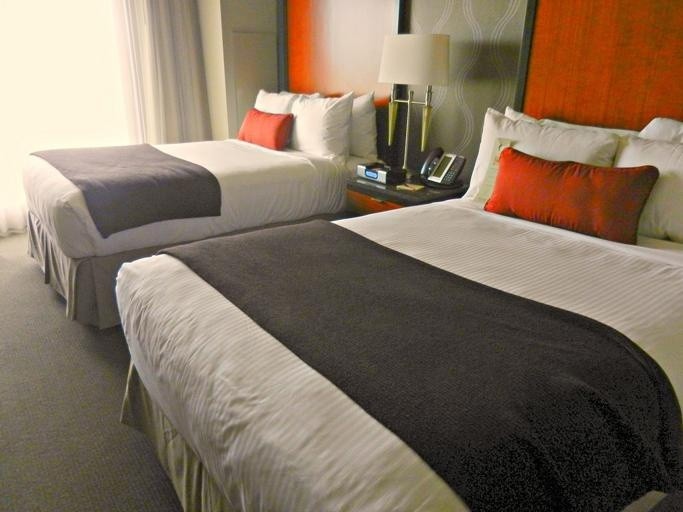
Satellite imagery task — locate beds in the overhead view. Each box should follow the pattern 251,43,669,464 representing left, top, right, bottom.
116,200,683,512
22,138,385,331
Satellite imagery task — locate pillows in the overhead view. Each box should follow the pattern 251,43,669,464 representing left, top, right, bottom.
237,90,378,162
465,106,682,246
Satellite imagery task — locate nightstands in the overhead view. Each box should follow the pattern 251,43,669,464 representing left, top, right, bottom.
346,180,468,215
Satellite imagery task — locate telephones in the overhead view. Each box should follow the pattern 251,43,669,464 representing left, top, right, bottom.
419,146,466,189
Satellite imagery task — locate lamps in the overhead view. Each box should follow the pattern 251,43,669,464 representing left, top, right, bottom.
378,34,450,179
